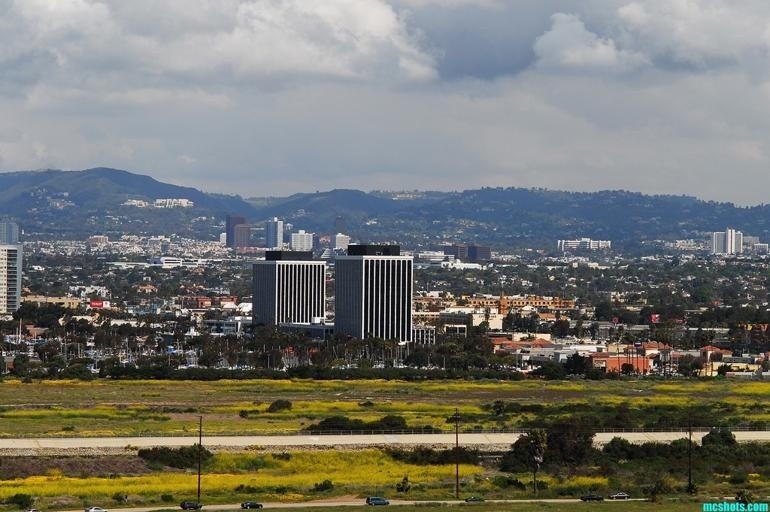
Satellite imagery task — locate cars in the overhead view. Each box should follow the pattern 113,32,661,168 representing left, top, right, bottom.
85,505,106,512
608,492,631,500
466,496,484,502
580,492,603,501
26,508,40,512
242,501,263,509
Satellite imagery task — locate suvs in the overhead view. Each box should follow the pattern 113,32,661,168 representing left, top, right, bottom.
180,500,201,510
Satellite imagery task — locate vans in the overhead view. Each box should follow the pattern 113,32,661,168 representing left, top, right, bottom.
365,496,390,507
